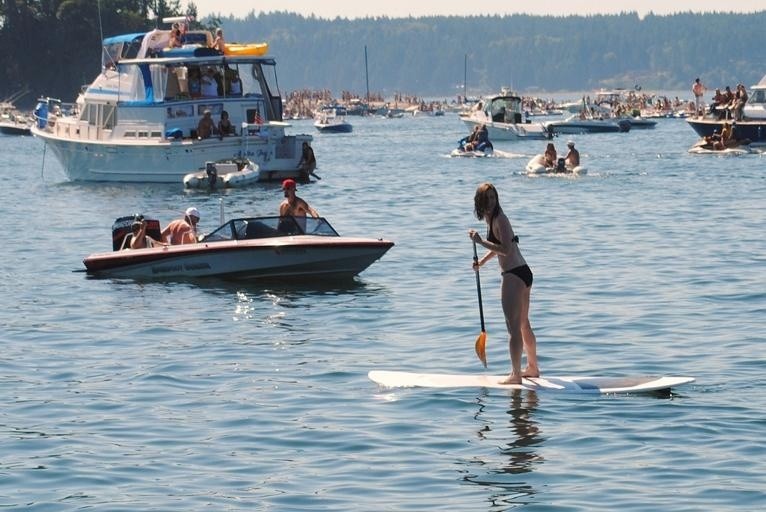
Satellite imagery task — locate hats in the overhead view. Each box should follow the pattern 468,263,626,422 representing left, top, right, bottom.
565,140,576,146
282,179,298,192
185,207,200,219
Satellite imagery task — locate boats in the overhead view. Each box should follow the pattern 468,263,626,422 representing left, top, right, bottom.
182,153,260,195
0,96,76,143
70,205,399,287
29,11,316,184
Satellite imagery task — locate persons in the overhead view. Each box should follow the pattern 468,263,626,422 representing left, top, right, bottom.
456,92,560,114
129,219,169,247
279,178,321,233
282,88,450,124
161,207,200,245
460,124,490,154
544,141,579,174
169,23,238,100
692,76,749,149
578,84,695,120
296,141,320,180
194,110,233,138
468,181,539,384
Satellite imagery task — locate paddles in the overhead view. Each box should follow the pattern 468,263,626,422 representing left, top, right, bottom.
472,239,486,367
